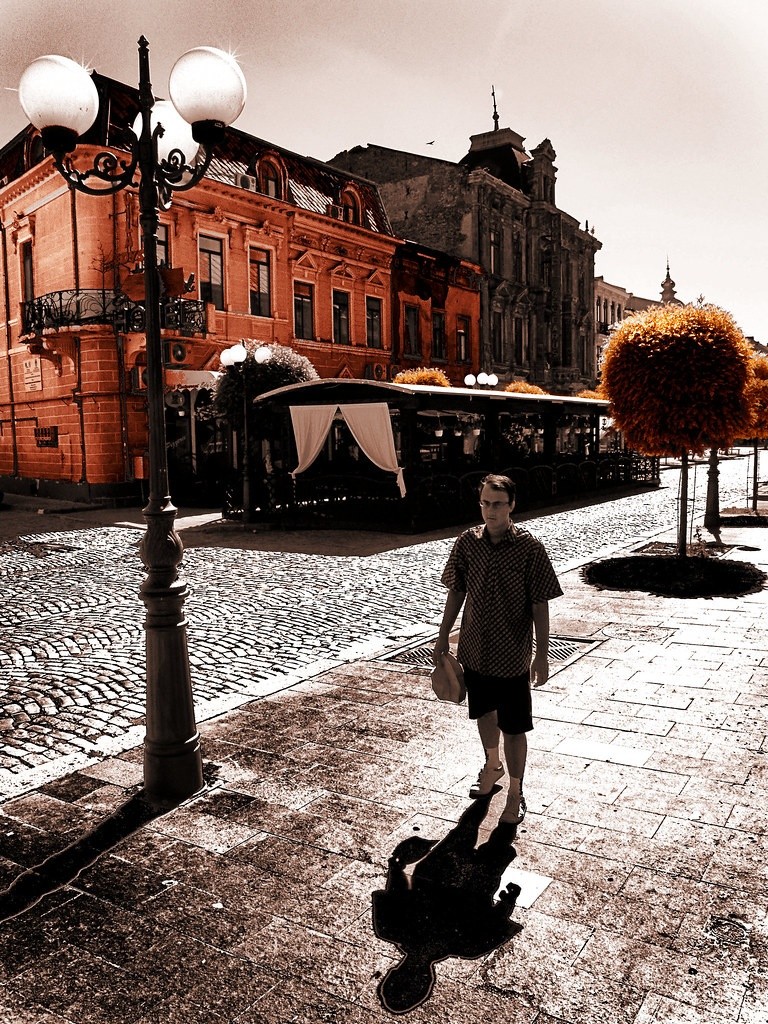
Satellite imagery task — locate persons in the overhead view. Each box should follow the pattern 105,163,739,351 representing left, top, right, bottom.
432,474,565,823
464,426,478,455
454,427,461,436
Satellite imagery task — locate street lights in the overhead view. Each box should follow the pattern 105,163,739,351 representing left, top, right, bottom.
19,33,249,803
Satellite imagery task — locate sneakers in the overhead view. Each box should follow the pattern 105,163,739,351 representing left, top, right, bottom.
500,794,527,823
470,760,505,795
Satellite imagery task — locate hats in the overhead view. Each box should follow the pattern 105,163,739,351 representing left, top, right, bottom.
431,652,466,704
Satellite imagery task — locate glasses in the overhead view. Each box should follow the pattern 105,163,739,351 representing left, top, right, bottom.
478,501,509,509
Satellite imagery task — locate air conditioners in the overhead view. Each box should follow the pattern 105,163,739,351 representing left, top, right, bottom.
129,365,150,392
163,339,194,364
